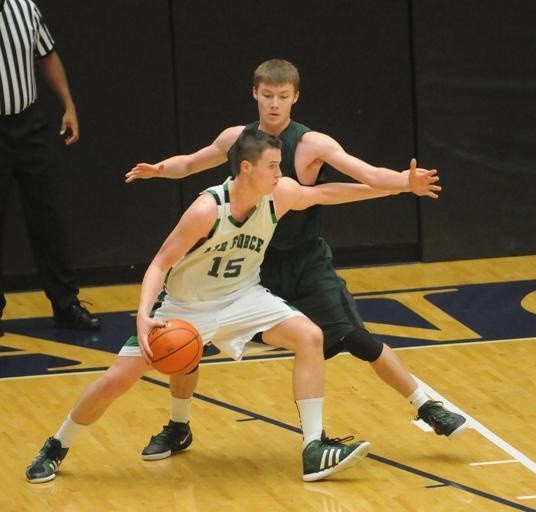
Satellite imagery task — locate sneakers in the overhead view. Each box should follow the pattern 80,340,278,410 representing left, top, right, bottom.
302,430,370,482
24,436,69,483
141,420,192,462
417,399,466,437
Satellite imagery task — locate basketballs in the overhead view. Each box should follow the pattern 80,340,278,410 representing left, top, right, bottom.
148,318,203,375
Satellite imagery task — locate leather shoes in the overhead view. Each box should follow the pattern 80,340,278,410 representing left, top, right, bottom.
54,304,103,330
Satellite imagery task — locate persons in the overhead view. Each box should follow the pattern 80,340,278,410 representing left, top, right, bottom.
123,56,469,461
0,0,102,337
24,130,432,484
